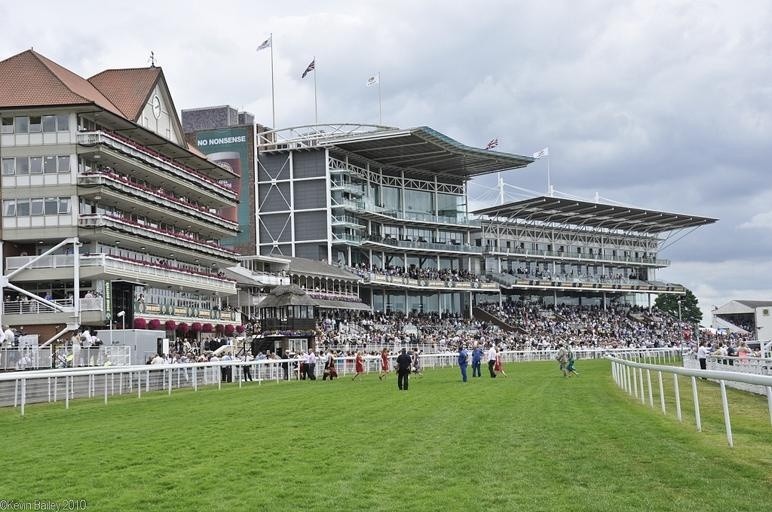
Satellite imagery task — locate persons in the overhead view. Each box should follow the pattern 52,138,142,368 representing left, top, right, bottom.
555,344,580,379
2,289,104,372
681,338,751,382
502,265,638,283
480,300,759,361
323,307,524,365
395,348,412,390
352,260,493,288
137,294,363,382
351,347,423,381
457,341,507,383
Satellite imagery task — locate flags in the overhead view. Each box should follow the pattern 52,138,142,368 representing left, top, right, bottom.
301,59,314,80
256,35,271,52
365,72,379,88
